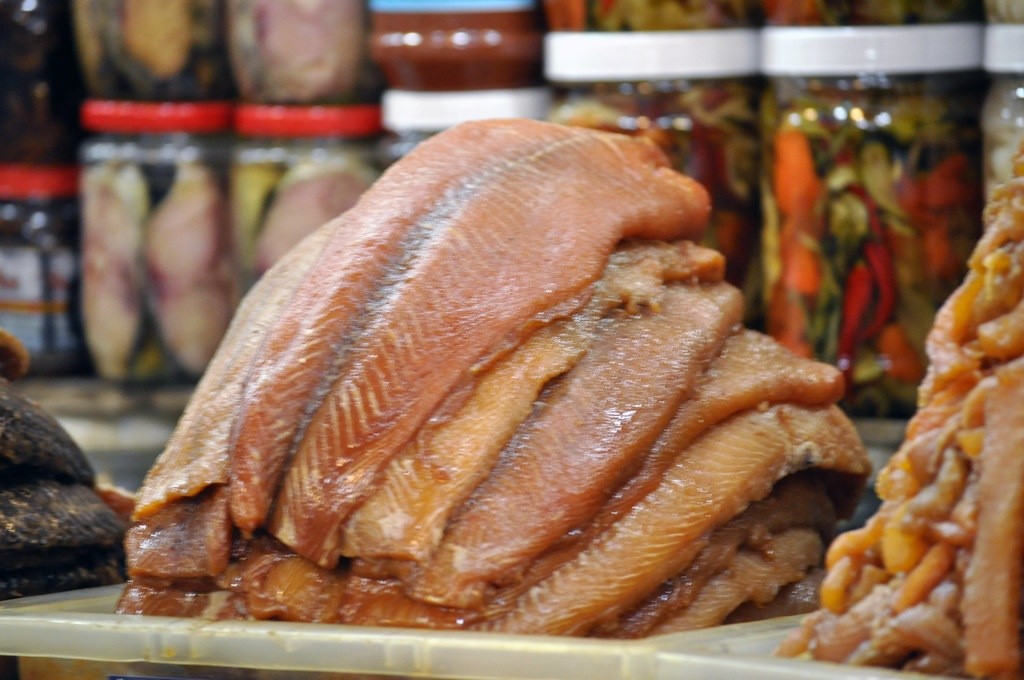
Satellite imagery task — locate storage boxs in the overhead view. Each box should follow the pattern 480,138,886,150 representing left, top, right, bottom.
0,580,966,680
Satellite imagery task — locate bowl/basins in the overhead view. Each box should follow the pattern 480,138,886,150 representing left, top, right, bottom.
1,581,916,680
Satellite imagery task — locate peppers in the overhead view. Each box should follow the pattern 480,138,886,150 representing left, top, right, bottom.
822,133,935,397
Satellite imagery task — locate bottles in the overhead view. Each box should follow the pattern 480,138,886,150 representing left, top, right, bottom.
2,1,1024,449
